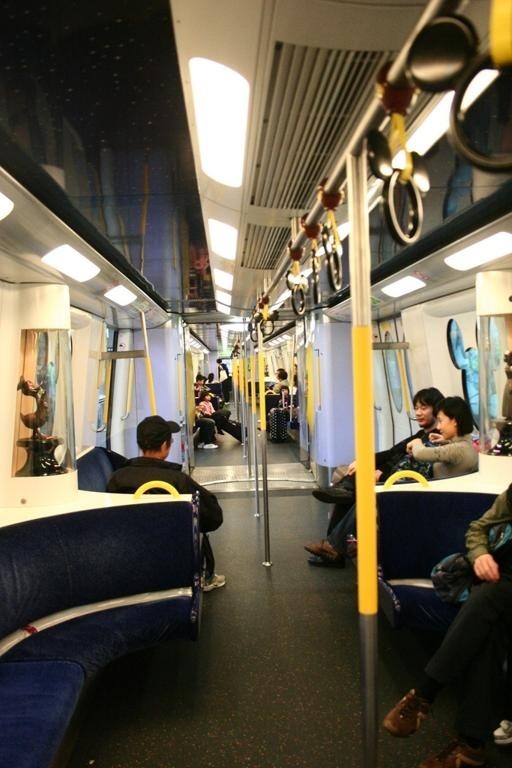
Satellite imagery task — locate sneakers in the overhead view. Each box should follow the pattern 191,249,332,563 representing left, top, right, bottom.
416,738,493,768
312,482,355,506
203,575,225,592
303,539,346,568
197,441,219,450
383,688,431,738
493,720,511,745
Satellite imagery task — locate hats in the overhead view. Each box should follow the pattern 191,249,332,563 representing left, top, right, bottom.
137,416,181,450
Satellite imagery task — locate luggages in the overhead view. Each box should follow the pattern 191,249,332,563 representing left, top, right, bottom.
268,393,288,443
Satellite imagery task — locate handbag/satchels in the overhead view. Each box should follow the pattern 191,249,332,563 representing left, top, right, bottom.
430,553,474,606
219,370,228,383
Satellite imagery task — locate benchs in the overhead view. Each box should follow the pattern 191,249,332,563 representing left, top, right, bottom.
76,445,183,492
376,492,502,695
0,499,201,767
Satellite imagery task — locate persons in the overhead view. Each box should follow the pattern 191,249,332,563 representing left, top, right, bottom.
194,358,309,450
307,387,445,568
502,352,512,422
493,657,511,744
304,396,479,561
383,482,512,768
106,415,226,592
